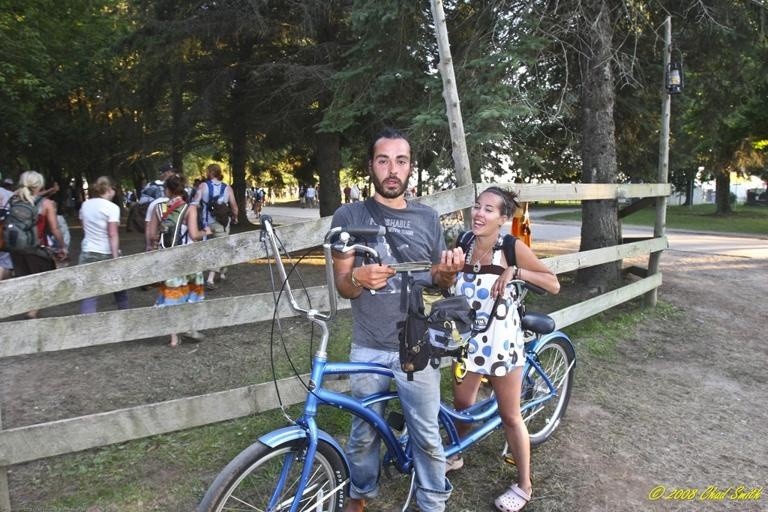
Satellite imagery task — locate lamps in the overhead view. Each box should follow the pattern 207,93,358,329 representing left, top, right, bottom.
662,42,684,95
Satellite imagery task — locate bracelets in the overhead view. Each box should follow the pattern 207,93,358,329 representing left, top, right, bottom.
511,264,518,279
351,269,362,288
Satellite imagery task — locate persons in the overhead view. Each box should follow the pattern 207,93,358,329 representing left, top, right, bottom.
450,187,560,512
330,130,467,512
343,182,367,204
410,187,415,197
0,160,320,347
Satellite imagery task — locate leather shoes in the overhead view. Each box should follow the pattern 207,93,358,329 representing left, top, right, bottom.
346,499,369,512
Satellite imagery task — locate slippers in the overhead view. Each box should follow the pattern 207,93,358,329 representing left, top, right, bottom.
495,484,533,511
168,337,182,348
181,332,206,341
445,457,464,474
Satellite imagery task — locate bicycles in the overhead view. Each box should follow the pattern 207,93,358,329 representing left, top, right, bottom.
192,209,582,511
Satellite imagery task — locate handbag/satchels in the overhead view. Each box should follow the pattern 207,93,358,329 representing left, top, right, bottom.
160,206,181,249
208,196,231,226
400,280,475,372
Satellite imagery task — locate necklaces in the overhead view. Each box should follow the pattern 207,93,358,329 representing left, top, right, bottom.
472,235,498,273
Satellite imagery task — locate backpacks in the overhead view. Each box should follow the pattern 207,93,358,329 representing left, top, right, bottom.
0,195,45,254
